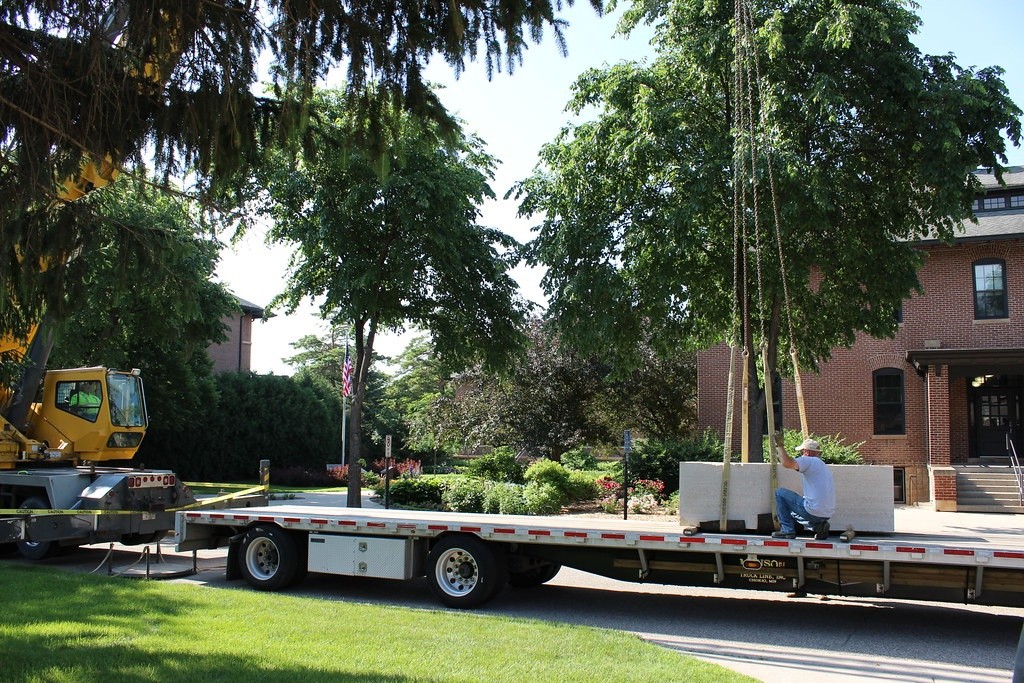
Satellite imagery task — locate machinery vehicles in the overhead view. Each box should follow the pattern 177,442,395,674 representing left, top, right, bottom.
0,0,271,561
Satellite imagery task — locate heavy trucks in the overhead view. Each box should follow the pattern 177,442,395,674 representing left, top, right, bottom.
175,504,1024,610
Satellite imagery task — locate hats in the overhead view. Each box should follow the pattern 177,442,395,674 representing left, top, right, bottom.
795,439,822,451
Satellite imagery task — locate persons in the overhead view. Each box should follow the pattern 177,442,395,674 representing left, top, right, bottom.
70,381,101,416
772,431,836,540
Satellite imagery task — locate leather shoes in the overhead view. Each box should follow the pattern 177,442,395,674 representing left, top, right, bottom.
772,531,796,539
814,521,830,539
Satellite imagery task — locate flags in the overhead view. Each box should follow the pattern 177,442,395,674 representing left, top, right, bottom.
342,342,352,398
